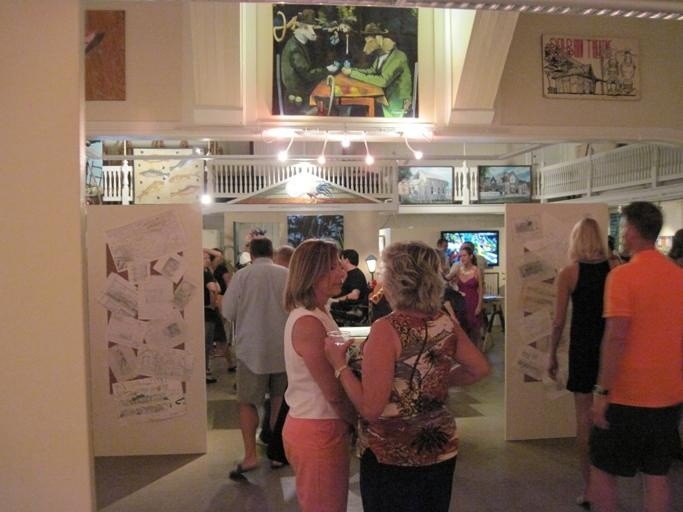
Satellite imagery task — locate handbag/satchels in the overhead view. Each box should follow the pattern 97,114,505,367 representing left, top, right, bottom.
265,382,289,466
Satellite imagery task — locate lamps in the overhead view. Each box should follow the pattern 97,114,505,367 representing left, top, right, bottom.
364,252,378,281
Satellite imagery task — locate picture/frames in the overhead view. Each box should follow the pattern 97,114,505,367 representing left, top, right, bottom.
540,32,640,102
395,164,455,206
476,162,531,204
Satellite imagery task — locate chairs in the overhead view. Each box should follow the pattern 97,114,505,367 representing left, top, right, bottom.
328,287,373,327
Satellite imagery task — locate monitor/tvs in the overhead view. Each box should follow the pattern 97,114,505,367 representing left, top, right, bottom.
441,230,500,266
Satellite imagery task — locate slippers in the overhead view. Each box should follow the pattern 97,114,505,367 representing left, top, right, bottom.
229,464,257,477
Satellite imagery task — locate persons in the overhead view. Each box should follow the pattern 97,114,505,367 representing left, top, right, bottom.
270,238,361,511
546,218,628,507
583,200,683,511
324,241,491,511
203,239,488,481
607,233,631,268
667,228,683,261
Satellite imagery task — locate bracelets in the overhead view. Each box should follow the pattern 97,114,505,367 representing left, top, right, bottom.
591,384,609,399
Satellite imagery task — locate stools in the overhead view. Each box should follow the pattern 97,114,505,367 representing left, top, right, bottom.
479,294,504,339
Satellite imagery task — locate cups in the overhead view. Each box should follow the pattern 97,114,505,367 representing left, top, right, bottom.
327,330,350,361
